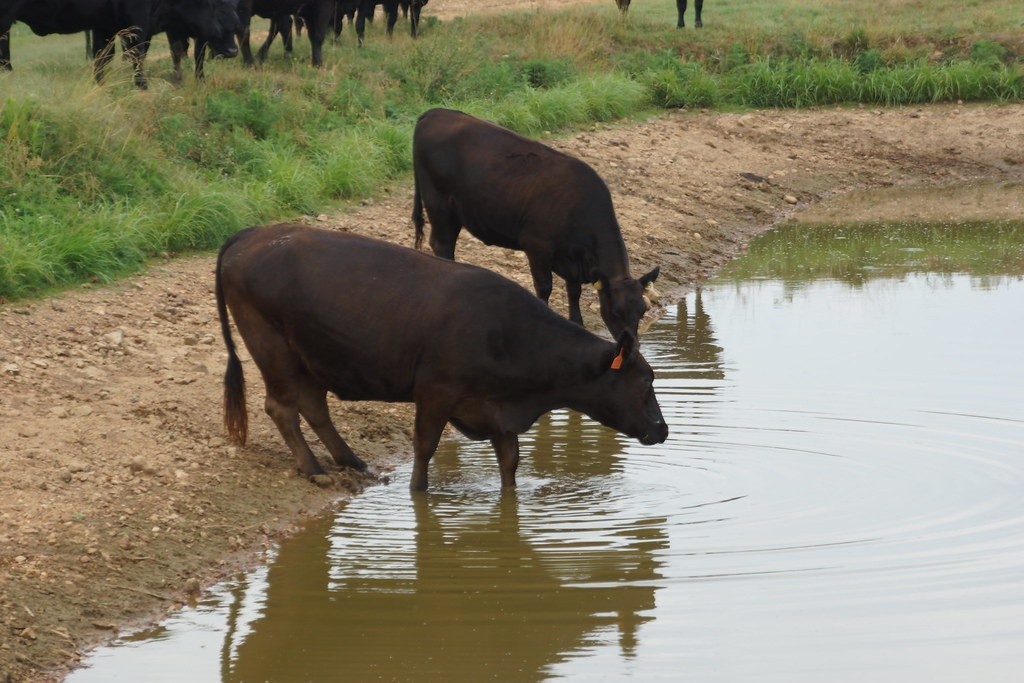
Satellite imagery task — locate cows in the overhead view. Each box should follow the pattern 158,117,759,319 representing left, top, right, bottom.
614,0,706,29
0,0,430,92
214,224,671,495
410,107,660,352
